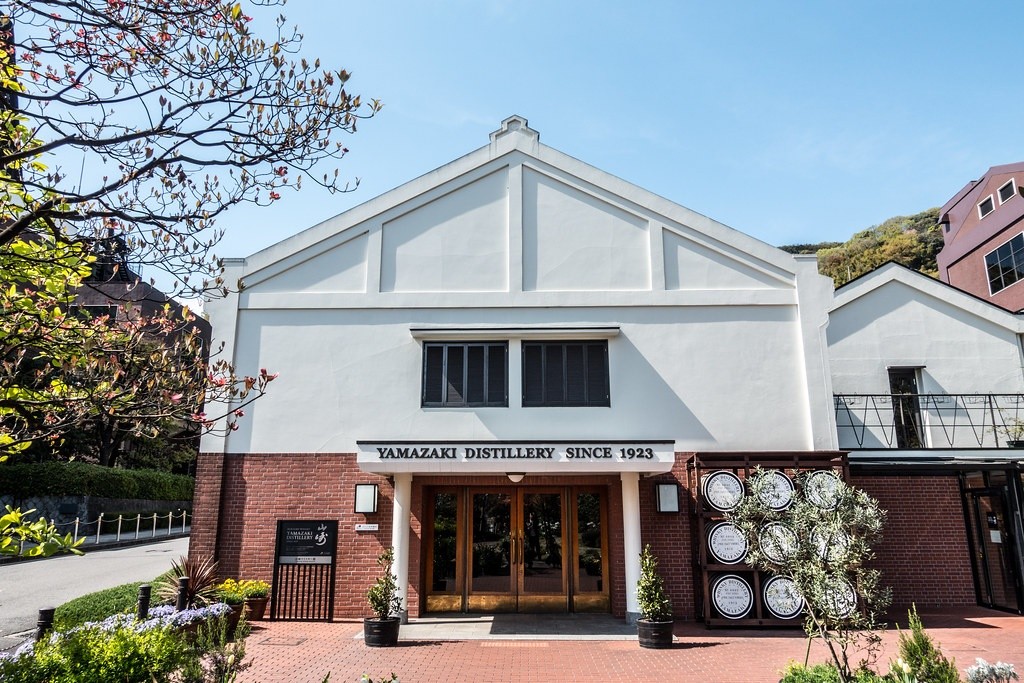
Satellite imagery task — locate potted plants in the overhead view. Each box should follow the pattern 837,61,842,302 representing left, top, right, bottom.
985,415,1024,449
363,547,404,648
634,543,675,650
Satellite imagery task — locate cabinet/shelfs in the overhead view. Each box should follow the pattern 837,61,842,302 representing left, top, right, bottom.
685,451,867,630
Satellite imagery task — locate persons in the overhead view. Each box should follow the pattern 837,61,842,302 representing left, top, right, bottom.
525,520,562,567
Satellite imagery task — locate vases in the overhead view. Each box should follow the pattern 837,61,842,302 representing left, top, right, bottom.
244,598,268,621
226,604,242,638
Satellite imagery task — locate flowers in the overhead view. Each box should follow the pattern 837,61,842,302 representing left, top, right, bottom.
216,578,272,606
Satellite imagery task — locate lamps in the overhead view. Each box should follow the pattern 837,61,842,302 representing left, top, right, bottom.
506,472,526,483
354,484,378,514
655,483,680,513
